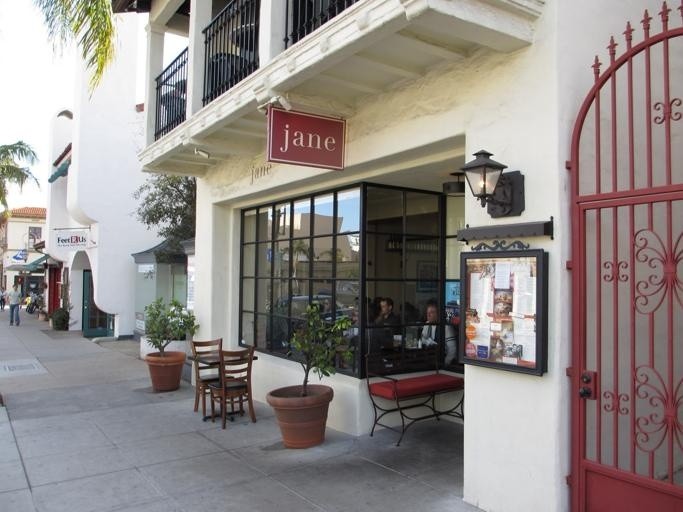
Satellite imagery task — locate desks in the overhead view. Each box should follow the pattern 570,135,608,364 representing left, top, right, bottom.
186,352,258,422
173,79,187,96
227,23,258,53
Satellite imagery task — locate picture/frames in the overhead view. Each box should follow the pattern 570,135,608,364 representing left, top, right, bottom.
459,249,548,376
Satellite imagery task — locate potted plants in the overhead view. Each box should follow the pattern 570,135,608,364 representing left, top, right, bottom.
265,305,353,448
143,296,199,393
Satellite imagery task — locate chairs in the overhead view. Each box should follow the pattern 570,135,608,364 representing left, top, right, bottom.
190,338,257,429
159,90,185,133
207,52,258,102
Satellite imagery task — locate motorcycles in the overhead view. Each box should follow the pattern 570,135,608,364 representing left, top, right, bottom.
23,287,44,313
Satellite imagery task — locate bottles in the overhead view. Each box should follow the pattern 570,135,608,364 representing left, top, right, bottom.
413,337,423,348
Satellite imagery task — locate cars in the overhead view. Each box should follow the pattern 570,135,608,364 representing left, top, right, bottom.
263,292,353,350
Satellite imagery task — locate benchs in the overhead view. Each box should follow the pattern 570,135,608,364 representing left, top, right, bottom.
364,348,463,447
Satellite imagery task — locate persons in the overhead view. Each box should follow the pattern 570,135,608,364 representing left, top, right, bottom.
414,300,457,368
371,298,397,329
1,287,5,311
320,294,336,319
5,283,22,326
367,303,394,367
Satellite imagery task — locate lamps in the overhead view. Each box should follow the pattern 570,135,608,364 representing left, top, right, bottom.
460,149,525,218
